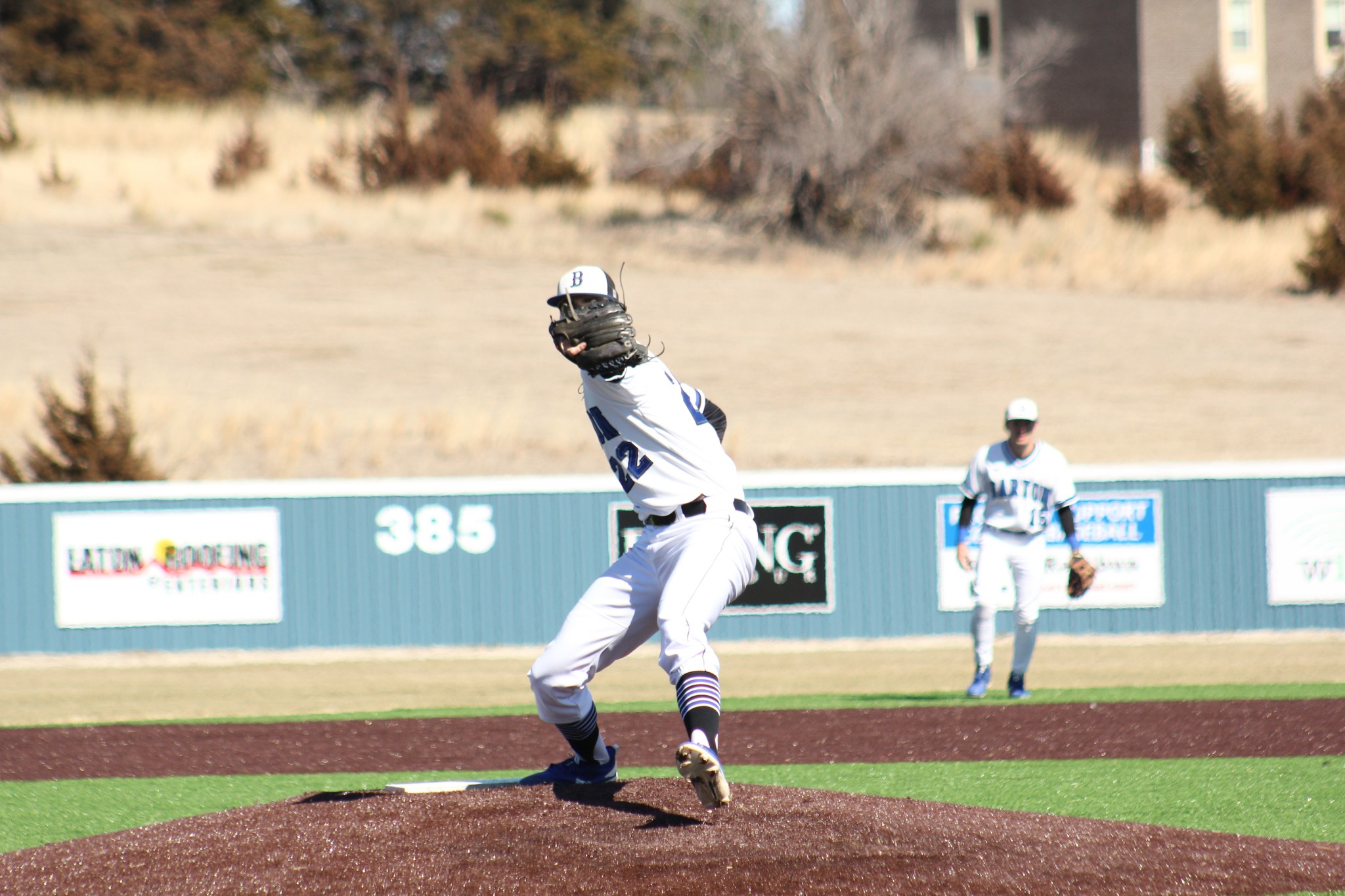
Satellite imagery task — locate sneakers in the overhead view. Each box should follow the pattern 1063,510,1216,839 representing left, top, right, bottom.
518,745,618,785
675,742,730,807
1007,670,1025,699
967,666,990,698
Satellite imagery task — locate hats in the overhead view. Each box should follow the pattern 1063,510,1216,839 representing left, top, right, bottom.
547,265,619,307
1006,398,1038,422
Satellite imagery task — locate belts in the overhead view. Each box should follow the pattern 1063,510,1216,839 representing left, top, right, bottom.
645,499,749,526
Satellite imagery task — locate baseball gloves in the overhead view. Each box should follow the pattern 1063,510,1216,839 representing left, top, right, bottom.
1067,550,1096,598
548,259,666,379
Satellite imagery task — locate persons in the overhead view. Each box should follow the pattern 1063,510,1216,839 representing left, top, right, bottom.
520,261,759,810
957,397,1082,702
